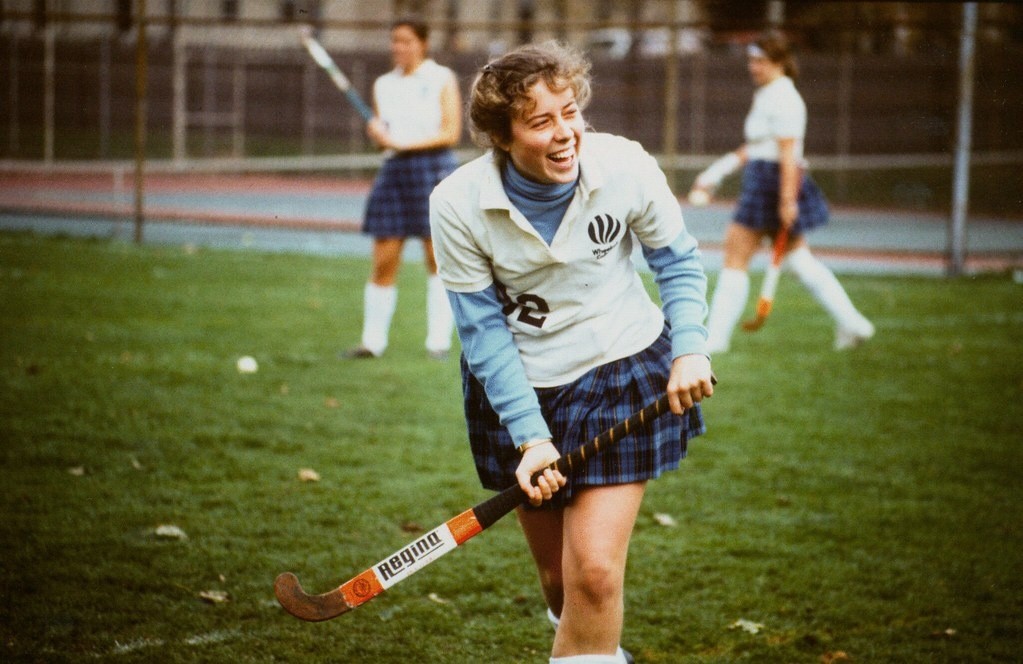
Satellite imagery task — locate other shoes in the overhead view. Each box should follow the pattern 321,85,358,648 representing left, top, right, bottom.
340,347,373,359
833,319,875,352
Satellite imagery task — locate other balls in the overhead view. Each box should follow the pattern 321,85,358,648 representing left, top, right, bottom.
234,354,260,375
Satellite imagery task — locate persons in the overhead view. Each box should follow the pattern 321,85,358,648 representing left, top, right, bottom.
688,29,875,356
430,39,712,664
341,20,462,359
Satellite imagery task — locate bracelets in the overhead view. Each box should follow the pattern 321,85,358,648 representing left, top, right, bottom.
518,438,551,452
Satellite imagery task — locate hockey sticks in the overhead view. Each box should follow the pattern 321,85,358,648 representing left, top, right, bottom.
740,158,811,333
297,23,381,125
269,369,720,624
